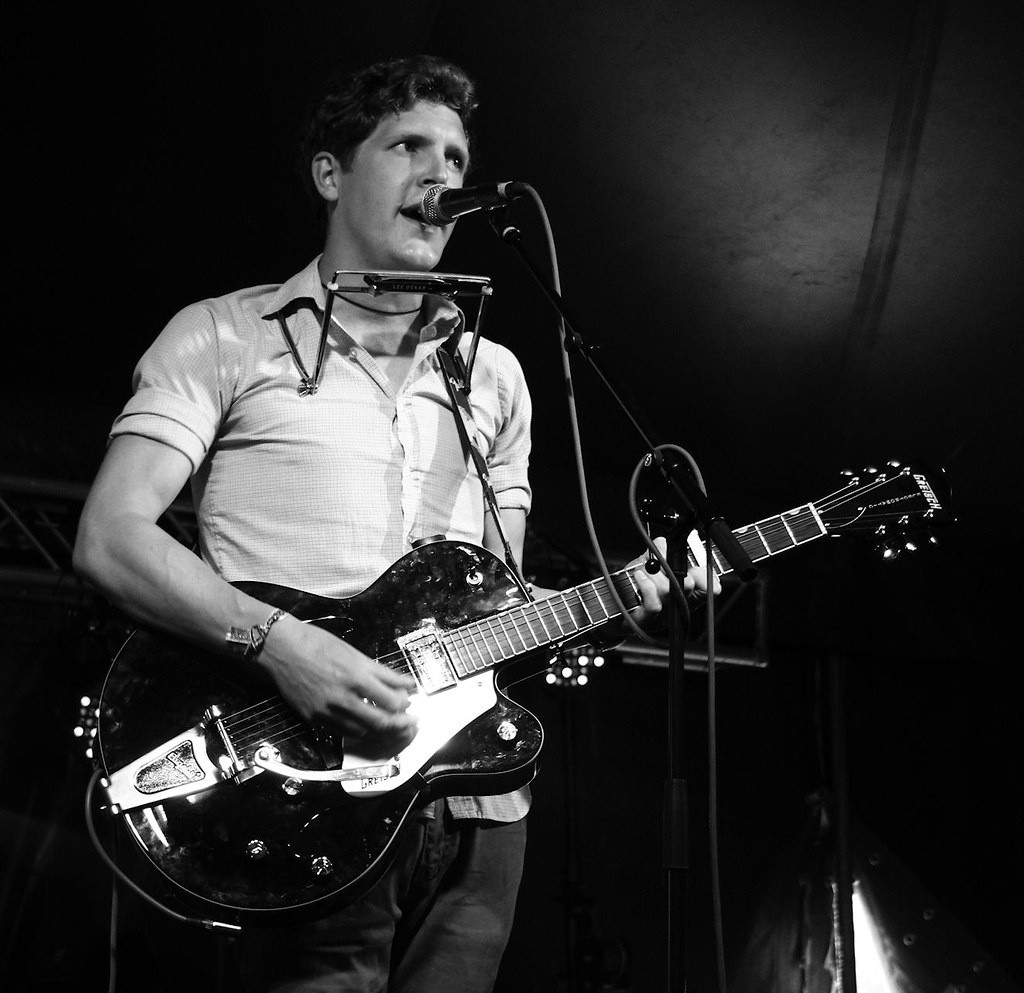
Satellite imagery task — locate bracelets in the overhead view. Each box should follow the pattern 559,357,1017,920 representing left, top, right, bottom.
225,610,287,665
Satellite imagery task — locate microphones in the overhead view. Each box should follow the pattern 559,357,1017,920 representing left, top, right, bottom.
420,181,529,227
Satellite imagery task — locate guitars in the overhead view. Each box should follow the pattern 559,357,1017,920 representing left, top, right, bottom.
90,456,968,930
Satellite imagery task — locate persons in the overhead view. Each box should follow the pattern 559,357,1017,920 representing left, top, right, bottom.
66,62,725,993
